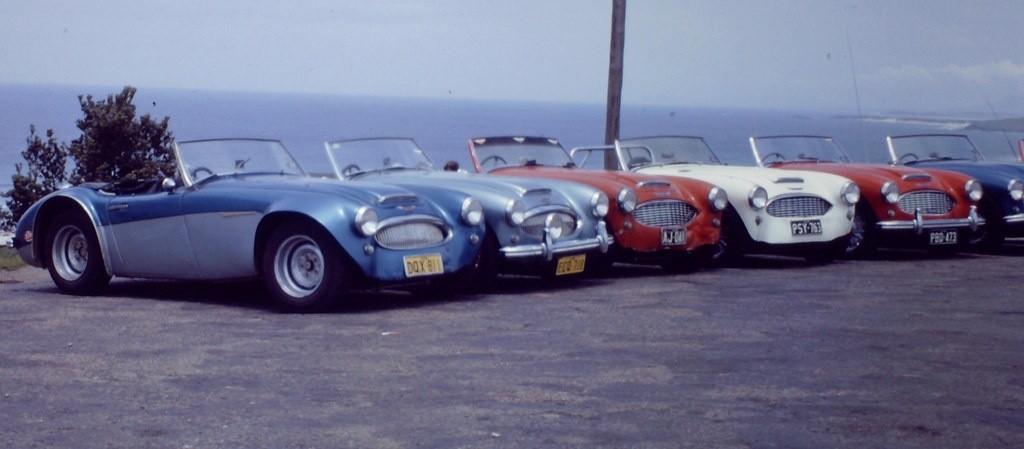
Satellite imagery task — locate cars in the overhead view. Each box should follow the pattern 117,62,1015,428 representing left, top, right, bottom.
748,138,988,249
305,138,615,282
457,137,729,269
886,134,1024,251
12,136,488,310
570,137,860,264
